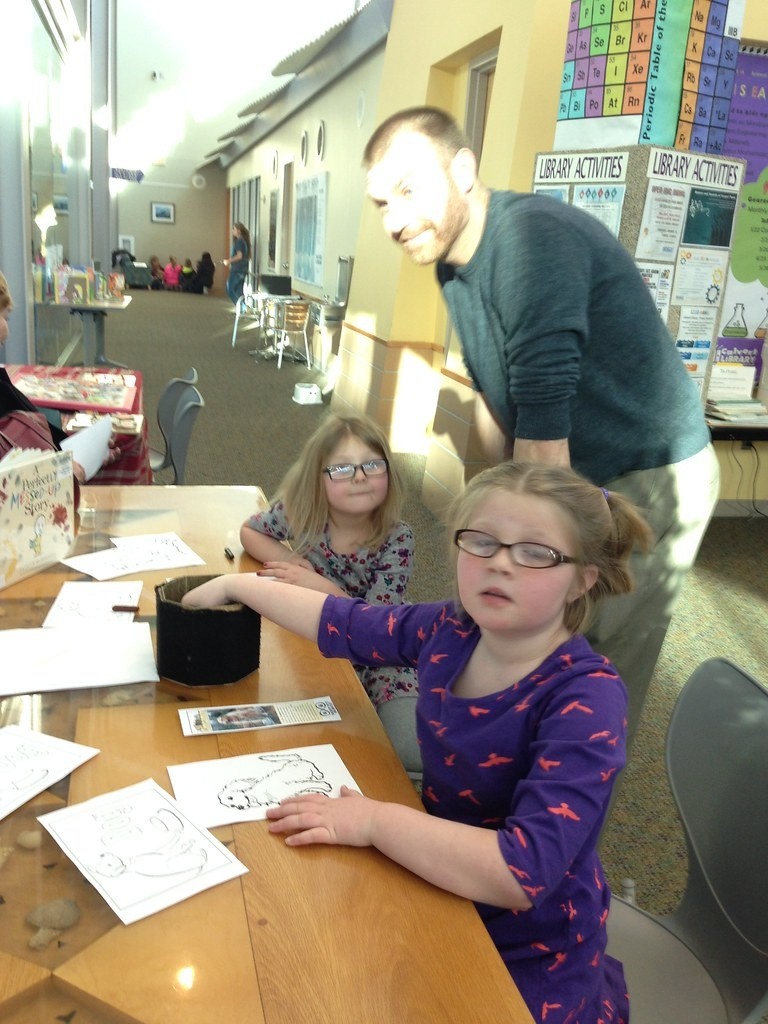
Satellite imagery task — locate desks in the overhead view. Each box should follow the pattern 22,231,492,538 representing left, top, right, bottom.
65,294,132,370
0,484,534,1024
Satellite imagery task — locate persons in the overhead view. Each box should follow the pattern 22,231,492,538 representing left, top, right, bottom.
179,258,197,289
224,222,251,317
181,463,655,1024
362,106,724,856
240,415,421,771
0,271,120,481
163,256,181,290
151,257,164,280
195,253,215,292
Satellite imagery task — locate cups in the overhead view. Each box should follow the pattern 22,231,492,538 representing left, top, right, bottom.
223,259,229,266
155,574,261,687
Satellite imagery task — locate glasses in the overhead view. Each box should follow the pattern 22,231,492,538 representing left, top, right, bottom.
455,529,574,569
323,459,387,480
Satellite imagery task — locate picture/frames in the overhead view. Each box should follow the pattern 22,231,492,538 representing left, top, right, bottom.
53,192,71,216
151,201,175,225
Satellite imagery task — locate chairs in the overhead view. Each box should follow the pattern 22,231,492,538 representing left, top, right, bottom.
170,385,205,484
606,656,767,1024
146,367,198,473
271,299,314,371
